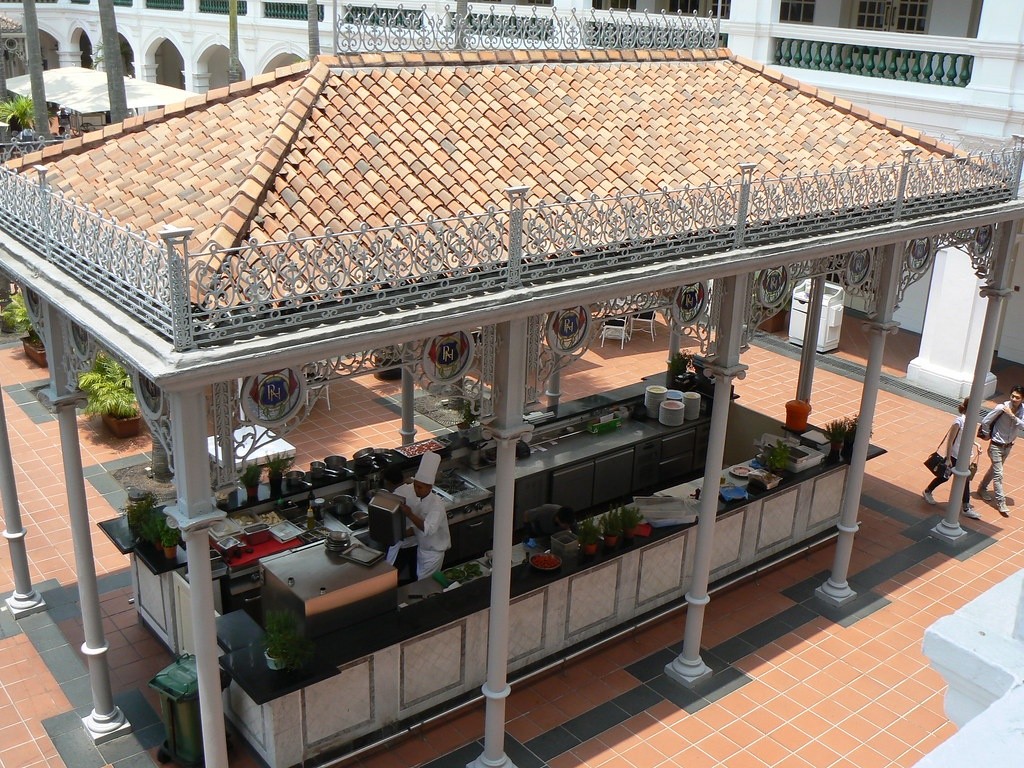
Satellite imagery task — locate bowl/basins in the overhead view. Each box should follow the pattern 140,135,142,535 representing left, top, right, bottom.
322,531,351,552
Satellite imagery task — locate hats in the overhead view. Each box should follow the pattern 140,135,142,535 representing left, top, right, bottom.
412,452,442,485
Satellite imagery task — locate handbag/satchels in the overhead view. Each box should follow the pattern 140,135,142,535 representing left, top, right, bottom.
924,452,952,480
976,404,1006,439
965,455,979,480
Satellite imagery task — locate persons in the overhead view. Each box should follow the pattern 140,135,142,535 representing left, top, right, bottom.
391,450,451,582
921,397,982,518
977,384,1024,512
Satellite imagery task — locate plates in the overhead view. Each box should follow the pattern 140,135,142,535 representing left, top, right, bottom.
530,552,563,571
442,568,467,581
393,439,446,459
729,466,754,477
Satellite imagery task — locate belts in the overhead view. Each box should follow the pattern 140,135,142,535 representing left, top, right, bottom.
989,440,1013,448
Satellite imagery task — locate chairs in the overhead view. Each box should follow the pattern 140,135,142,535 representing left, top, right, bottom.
301,362,333,416
601,311,629,349
627,302,656,342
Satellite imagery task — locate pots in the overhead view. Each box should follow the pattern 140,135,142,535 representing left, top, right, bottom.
324,456,354,475
346,511,369,528
353,447,392,468
367,488,389,500
309,461,340,479
329,494,358,514
284,470,313,489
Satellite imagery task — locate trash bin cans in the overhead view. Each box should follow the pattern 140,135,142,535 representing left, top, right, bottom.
146,653,234,766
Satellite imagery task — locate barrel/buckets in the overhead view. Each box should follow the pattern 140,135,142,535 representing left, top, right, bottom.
785,399,812,431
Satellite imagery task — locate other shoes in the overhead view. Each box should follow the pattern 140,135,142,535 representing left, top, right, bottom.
978,489,992,501
997,502,1009,513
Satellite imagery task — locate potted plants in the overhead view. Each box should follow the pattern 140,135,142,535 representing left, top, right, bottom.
143,515,165,551
749,276,794,331
452,396,482,437
157,519,182,558
579,517,601,555
603,509,624,546
75,351,142,437
2,286,49,367
844,418,871,445
825,422,849,451
268,452,294,492
243,461,260,499
258,606,315,670
767,439,791,478
620,503,642,541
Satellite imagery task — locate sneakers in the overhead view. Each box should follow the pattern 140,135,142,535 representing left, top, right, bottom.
962,508,981,517
922,489,936,505
